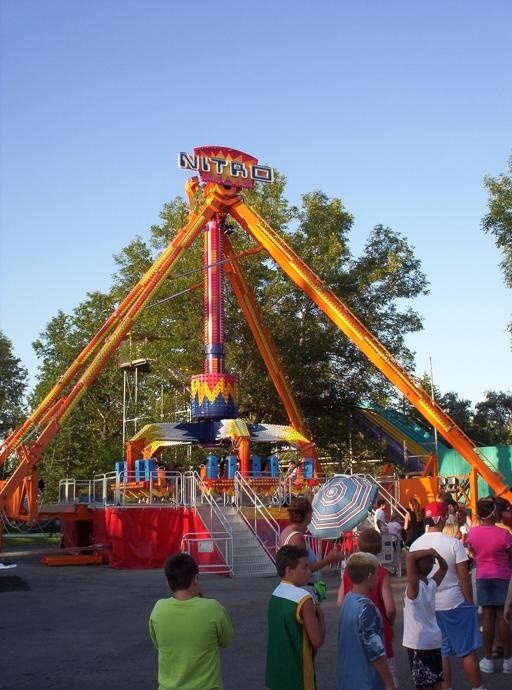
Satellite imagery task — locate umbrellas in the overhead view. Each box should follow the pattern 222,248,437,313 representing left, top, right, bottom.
304,474,377,541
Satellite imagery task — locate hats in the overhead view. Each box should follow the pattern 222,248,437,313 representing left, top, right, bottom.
424,502,449,519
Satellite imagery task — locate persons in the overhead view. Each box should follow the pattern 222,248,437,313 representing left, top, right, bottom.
338,528,395,683
276,461,298,490
147,552,233,690
384,515,404,552
404,488,511,674
400,547,450,690
407,500,488,690
263,543,325,690
373,499,386,538
336,550,395,690
278,495,344,660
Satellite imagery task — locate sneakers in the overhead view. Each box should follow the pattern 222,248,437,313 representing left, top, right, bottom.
479,656,495,673
503,657,512,674
